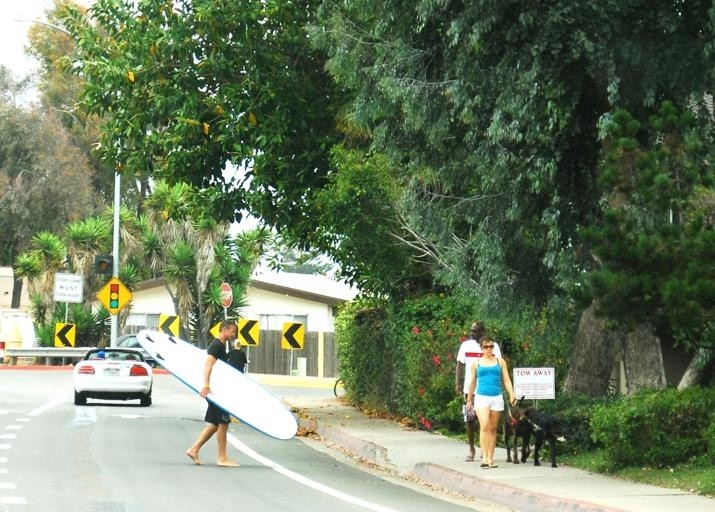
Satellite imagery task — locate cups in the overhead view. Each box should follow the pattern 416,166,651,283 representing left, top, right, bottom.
483,345,494,348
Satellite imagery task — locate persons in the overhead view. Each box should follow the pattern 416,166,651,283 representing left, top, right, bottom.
227,339,247,373
186,320,241,467
96,351,105,358
455,321,517,467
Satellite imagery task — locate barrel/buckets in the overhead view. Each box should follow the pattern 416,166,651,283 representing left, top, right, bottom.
481,462,498,468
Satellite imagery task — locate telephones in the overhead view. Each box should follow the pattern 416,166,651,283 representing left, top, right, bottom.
52,272,83,303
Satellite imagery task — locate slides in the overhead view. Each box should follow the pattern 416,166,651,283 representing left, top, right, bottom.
136,330,297,440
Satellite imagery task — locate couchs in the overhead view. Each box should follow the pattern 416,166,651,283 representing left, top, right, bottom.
109,284,118,309
93,255,113,274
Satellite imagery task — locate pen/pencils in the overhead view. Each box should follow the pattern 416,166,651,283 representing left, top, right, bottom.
0,308,39,364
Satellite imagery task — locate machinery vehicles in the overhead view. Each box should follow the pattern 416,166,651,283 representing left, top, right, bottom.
69,345,154,408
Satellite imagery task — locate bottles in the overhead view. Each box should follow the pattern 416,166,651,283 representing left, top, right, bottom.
234,339,241,349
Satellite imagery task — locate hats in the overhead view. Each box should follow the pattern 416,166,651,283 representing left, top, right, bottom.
107,334,159,370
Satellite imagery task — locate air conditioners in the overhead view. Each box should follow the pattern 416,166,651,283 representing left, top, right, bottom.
503,403,558,468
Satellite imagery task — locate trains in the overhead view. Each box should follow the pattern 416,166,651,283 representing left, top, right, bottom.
217,283,234,308
